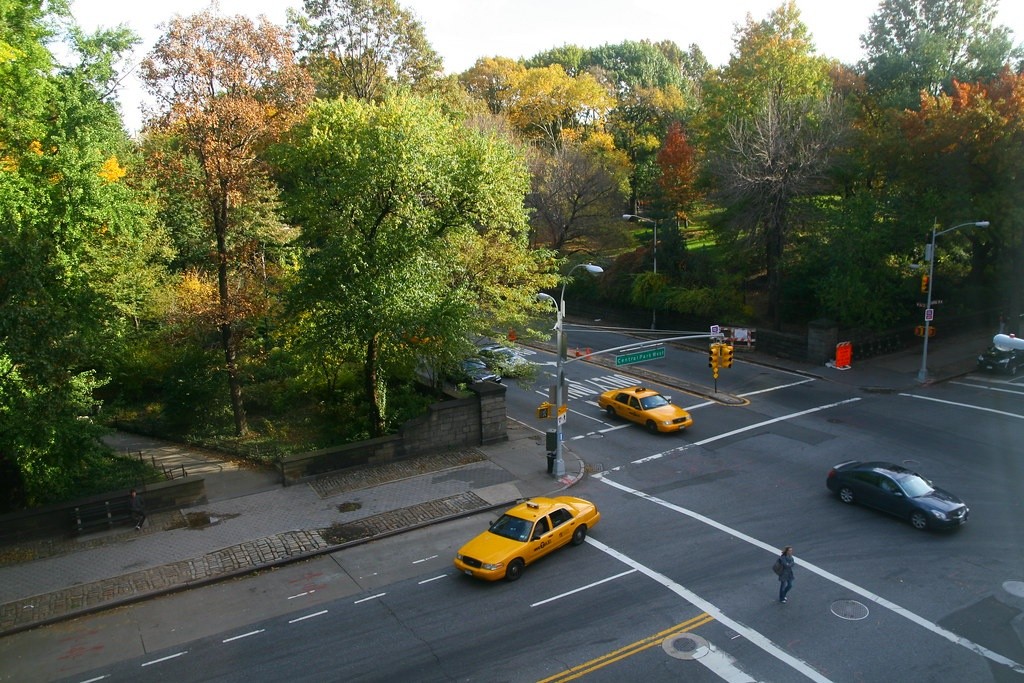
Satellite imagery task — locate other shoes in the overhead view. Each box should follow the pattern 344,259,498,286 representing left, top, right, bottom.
781,597,788,602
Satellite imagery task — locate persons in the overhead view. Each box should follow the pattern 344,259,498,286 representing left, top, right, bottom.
779,546,795,603
127,490,146,530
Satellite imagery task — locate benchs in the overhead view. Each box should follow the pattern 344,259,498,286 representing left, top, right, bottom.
71,496,131,537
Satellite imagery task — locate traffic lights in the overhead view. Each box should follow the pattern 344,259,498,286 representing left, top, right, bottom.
709,344,720,370
915,326,924,337
722,345,735,369
928,327,936,337
921,274,931,293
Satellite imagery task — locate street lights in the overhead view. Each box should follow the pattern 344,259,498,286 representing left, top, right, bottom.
915,216,990,385
622,213,658,330
536,263,603,473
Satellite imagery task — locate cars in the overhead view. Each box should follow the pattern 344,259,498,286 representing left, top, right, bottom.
455,495,601,583
461,359,503,383
976,347,1024,376
828,459,971,531
599,385,693,435
479,347,531,377
